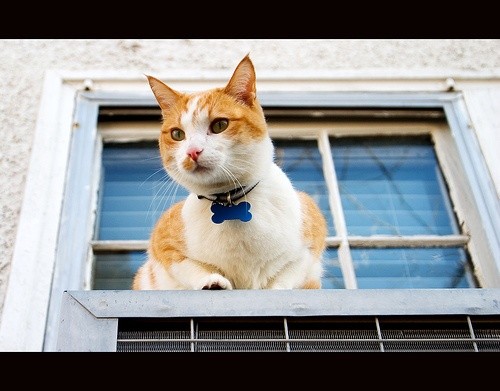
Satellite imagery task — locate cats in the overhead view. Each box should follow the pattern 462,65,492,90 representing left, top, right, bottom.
132,53,328,290
